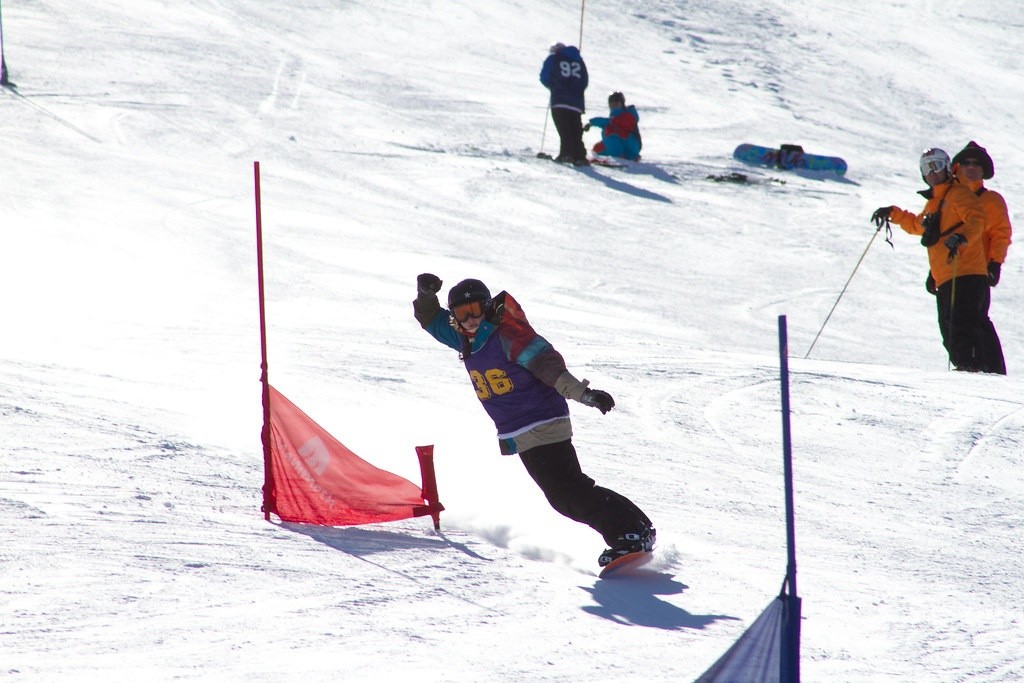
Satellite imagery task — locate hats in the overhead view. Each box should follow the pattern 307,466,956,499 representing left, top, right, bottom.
448,279,491,311
952,141,994,180
550,43,565,52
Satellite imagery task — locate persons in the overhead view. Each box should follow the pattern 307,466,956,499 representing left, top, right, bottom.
414,272,656,564
583,89,644,162
868,140,1011,376
540,42,591,165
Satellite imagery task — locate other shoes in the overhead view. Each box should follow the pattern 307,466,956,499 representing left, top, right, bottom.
598,520,656,567
555,152,591,166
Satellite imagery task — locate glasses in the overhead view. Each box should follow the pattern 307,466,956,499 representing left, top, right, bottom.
960,160,982,167
451,299,484,323
920,159,946,176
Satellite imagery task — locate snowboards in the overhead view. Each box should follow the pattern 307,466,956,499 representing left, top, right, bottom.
537,152,627,167
733,143,848,177
598,550,654,579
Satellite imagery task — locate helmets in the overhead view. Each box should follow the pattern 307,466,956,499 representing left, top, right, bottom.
919,147,950,172
608,92,625,102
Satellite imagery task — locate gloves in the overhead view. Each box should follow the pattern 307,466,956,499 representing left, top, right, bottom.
417,273,443,298
987,262,1001,287
580,387,615,415
925,270,938,296
870,207,890,227
943,232,968,264
583,123,591,132
